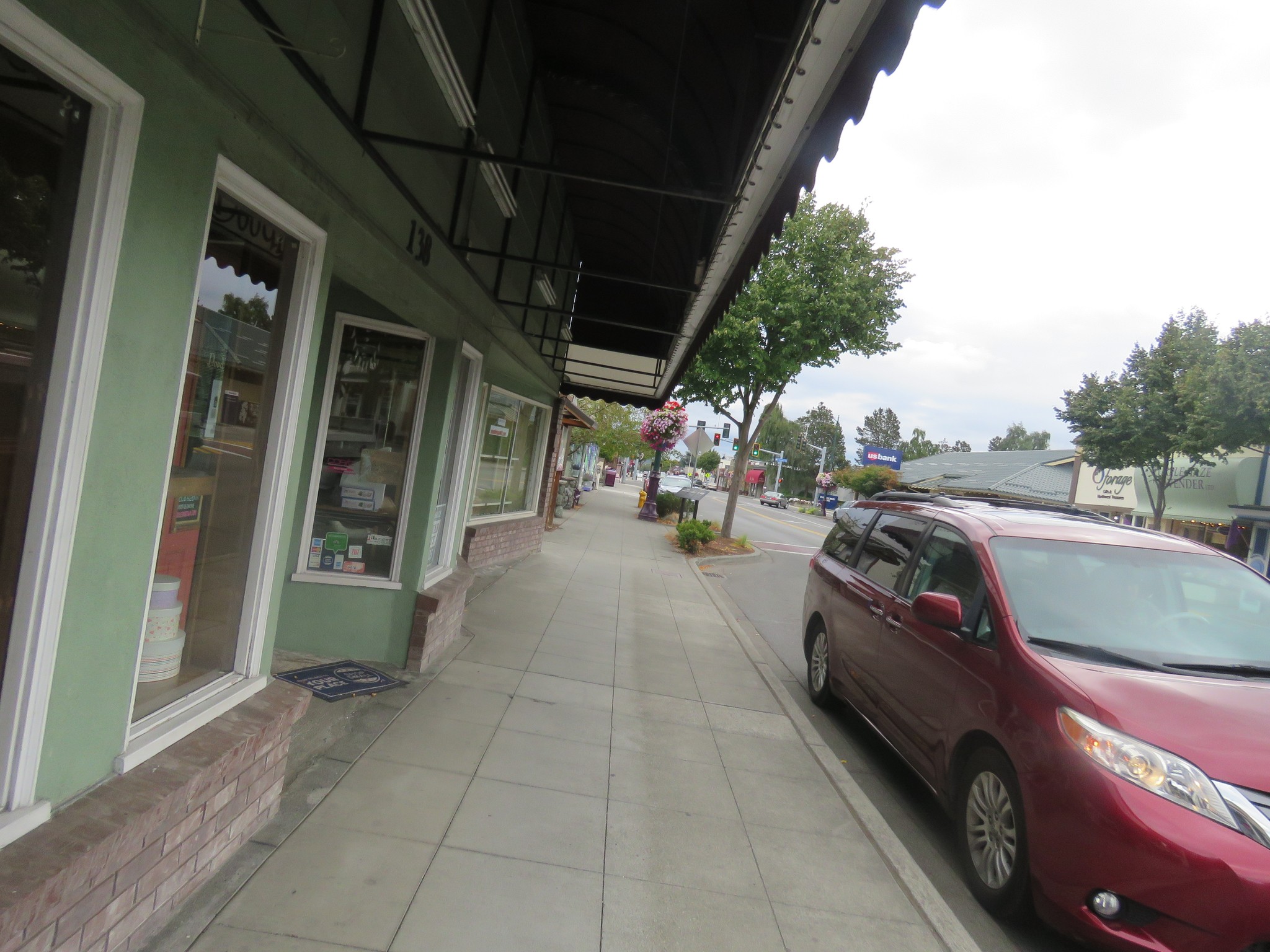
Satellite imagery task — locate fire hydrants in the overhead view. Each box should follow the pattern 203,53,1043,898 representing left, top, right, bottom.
638,490,647,508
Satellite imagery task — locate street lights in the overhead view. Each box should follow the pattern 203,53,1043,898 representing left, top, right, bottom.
811,409,840,516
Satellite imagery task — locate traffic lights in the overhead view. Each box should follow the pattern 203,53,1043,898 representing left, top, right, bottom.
751,443,760,457
779,479,783,483
801,439,807,454
796,433,803,452
713,433,721,446
732,438,739,451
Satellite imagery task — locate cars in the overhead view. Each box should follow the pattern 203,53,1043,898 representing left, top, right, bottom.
643,476,664,492
705,482,717,491
631,470,666,488
760,491,789,509
690,477,697,483
693,480,703,487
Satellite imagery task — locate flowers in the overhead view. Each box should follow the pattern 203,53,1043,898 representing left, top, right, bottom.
640,401,688,452
816,472,837,490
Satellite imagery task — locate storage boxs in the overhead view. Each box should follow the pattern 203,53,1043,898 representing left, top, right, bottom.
341,487,376,510
137,574,186,682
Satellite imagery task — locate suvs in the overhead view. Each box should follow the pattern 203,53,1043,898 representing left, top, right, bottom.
802,489,1270,952
702,480,711,489
832,500,856,523
656,474,699,506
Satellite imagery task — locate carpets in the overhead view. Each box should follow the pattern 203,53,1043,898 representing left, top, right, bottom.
272,659,410,703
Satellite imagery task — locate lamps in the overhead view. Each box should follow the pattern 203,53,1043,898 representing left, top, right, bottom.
561,322,573,341
477,142,517,217
535,272,556,305
397,0,477,131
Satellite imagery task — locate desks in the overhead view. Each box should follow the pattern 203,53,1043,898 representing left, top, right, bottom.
312,493,397,561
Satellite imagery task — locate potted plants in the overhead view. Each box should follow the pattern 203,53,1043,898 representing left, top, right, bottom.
583,473,593,487
573,464,580,476
